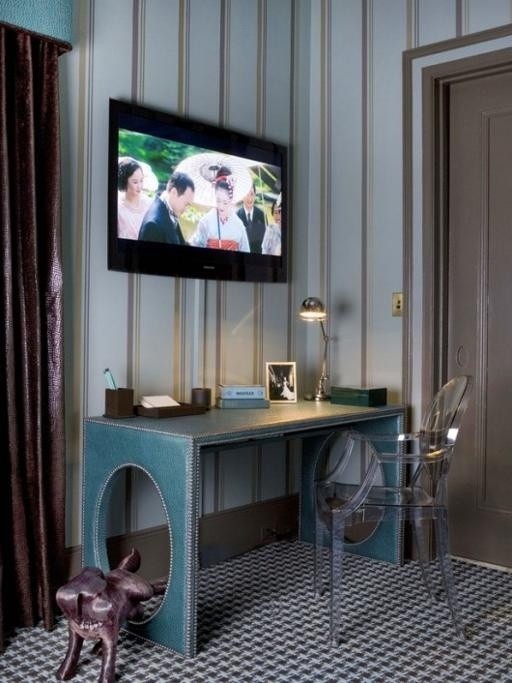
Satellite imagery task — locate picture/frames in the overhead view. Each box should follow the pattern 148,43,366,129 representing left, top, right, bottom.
265,361,297,404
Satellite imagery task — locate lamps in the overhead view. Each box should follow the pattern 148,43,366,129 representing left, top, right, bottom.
297,296,334,402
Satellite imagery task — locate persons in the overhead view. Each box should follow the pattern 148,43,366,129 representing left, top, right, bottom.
281,375,295,400
117,152,282,256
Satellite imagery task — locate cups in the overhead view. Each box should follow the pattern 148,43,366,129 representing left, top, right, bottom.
191,385,212,411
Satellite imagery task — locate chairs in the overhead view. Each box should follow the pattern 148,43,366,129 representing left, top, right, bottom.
313,374,475,649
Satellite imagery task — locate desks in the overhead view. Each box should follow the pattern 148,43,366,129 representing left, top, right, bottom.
82,393,406,660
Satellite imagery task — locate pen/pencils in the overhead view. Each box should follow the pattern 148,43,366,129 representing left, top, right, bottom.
103,370,112,390
105,368,117,390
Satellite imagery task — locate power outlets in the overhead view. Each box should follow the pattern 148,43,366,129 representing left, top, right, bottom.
392,292,403,317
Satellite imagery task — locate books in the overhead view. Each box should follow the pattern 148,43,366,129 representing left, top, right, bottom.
214,384,271,409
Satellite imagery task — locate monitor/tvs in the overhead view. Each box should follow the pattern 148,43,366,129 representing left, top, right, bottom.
108,96,290,284
